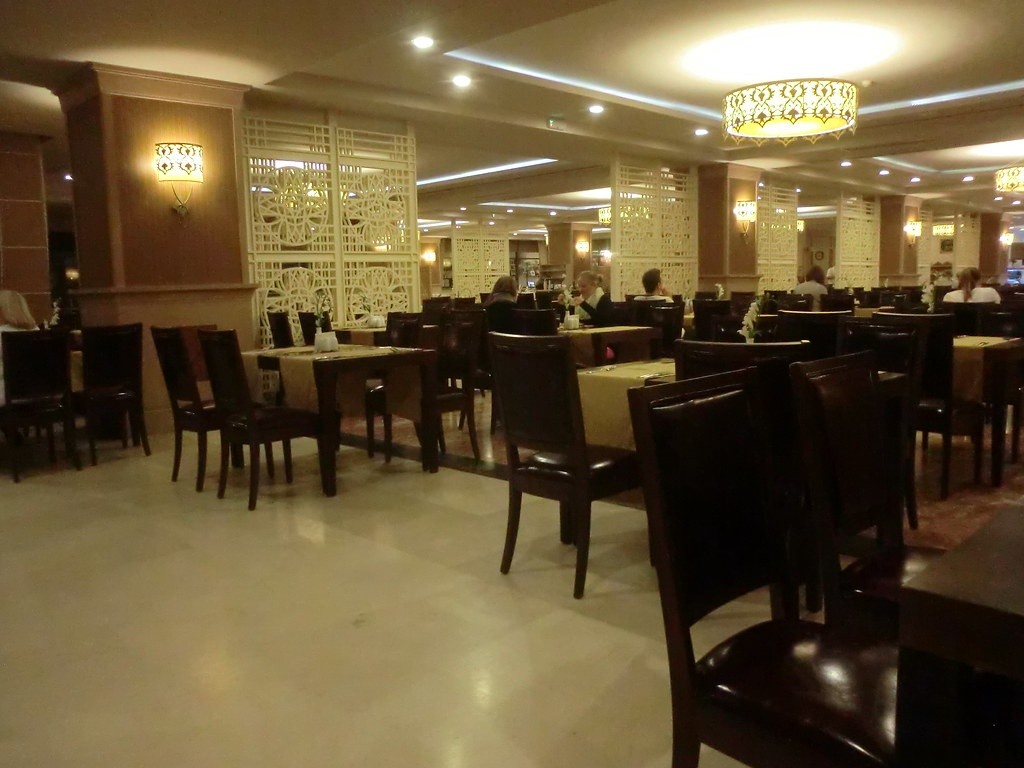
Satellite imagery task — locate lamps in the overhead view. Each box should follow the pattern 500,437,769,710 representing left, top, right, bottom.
732,200,757,238
994,165,1024,195
598,207,649,226
156,143,203,216
722,80,860,148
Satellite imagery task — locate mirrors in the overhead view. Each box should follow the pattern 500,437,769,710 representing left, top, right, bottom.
728,177,757,276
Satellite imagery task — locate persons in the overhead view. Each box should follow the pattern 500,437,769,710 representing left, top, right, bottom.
1014,272,1022,283
483,276,517,306
930,261,953,286
558,271,615,326
942,268,1001,304
794,266,828,307
632,269,675,303
826,267,835,285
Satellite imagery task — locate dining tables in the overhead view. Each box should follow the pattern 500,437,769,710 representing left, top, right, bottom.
553,324,663,367
230,344,439,496
325,323,437,414
0,323,122,440
561,357,910,574
952,334,1024,487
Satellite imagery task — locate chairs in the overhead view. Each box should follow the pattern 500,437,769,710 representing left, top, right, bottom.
627,365,898,767
72,322,152,466
199,329,325,510
488,330,644,599
0,328,83,483
149,325,274,493
267,282,1024,544
788,348,949,645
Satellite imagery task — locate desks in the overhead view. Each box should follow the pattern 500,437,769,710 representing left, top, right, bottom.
897,505,1024,768
